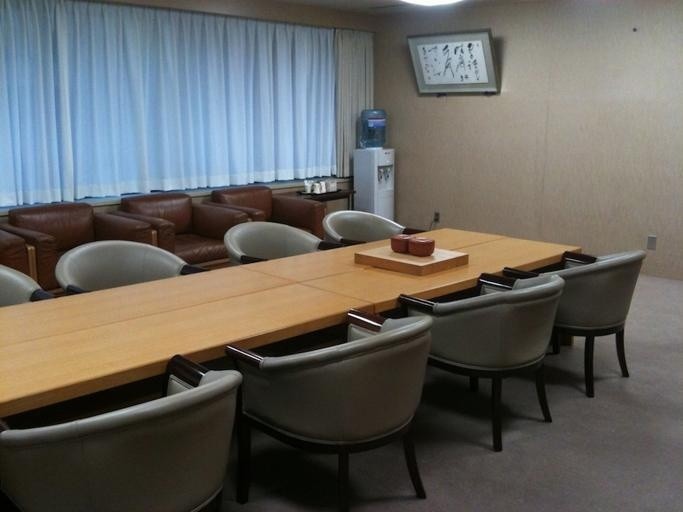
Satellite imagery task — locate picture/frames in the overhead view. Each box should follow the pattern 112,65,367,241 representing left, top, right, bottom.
406,28,501,96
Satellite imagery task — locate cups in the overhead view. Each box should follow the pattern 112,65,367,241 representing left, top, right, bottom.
303,179,337,194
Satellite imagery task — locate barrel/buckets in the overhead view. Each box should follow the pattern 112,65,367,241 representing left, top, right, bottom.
359,109,386,148
